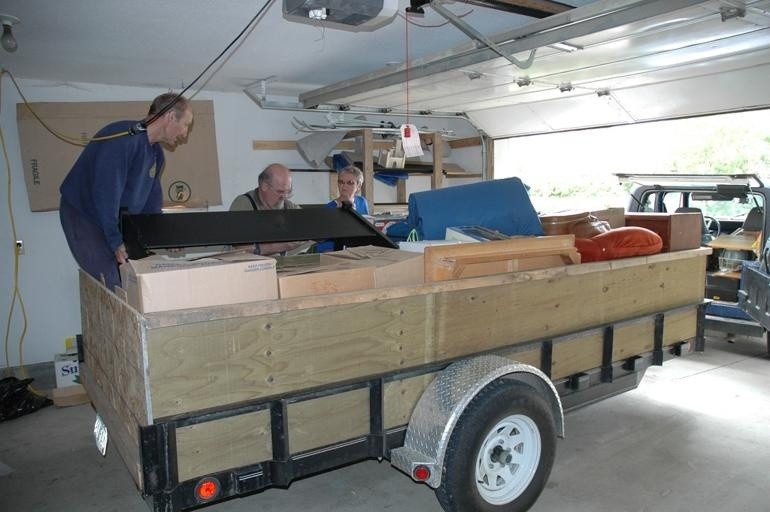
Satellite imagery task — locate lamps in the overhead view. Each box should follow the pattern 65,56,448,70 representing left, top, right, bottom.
0,14,22,53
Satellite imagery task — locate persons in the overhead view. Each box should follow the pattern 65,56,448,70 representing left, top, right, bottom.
228,162,306,255
58,91,195,294
315,164,370,252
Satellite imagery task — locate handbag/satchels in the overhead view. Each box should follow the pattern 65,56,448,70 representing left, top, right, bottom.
386,216,424,242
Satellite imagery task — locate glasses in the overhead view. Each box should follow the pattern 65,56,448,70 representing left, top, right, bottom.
337,180,355,186
270,188,294,200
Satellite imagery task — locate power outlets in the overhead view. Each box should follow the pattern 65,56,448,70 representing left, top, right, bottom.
16,239,26,256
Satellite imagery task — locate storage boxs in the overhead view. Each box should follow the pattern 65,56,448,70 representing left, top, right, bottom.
327,244,423,288
277,252,372,301
118,250,279,313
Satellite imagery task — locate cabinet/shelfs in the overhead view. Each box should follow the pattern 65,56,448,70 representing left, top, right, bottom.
623,211,703,251
328,128,441,212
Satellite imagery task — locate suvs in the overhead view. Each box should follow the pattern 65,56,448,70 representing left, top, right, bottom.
618,169,770,349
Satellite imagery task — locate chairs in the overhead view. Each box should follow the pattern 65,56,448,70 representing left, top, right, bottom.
674,206,712,241
743,207,763,230
